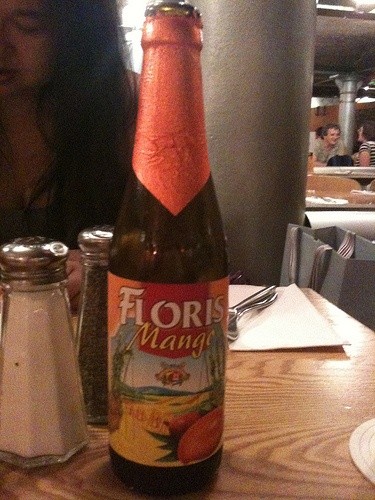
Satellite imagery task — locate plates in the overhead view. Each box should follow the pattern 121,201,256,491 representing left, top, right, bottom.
349,418,375,485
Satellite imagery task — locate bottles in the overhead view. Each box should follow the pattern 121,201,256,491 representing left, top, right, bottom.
1,236,93,468
106,0,232,493
75,228,116,427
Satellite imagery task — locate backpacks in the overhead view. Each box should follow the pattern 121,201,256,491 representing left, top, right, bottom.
327,155,354,166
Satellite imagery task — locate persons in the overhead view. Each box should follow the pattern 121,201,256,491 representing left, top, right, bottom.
354,121,375,167
313,124,345,166
1,0,142,313
309,127,324,151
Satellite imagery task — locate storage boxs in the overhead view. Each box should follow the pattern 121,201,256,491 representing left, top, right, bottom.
279,223,375,331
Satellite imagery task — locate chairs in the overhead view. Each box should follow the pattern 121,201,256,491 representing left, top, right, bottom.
305,175,362,200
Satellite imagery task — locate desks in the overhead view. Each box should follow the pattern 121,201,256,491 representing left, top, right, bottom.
0,286,375,500
313,166,375,179
